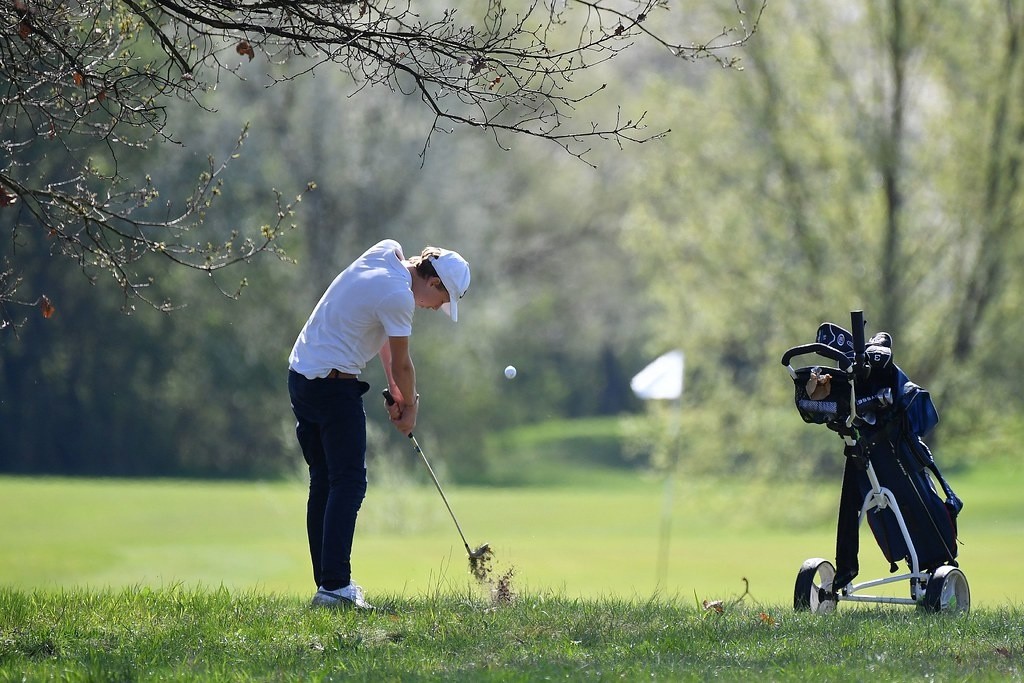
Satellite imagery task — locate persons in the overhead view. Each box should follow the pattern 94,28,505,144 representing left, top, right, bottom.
287,240,471,609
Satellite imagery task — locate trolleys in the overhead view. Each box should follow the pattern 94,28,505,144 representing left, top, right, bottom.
782,341,971,618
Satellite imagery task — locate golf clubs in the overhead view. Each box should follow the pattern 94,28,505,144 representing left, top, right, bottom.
381,387,491,562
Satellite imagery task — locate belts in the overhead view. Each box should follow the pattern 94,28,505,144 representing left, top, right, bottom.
328,369,359,378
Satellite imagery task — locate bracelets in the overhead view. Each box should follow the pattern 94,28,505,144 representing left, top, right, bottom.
406,394,419,409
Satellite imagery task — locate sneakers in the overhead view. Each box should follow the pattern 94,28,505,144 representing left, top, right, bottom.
312,580,376,609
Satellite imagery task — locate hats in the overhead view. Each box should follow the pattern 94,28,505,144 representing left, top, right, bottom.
422,247,470,323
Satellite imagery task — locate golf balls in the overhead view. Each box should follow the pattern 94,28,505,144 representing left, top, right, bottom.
503,365,517,380
